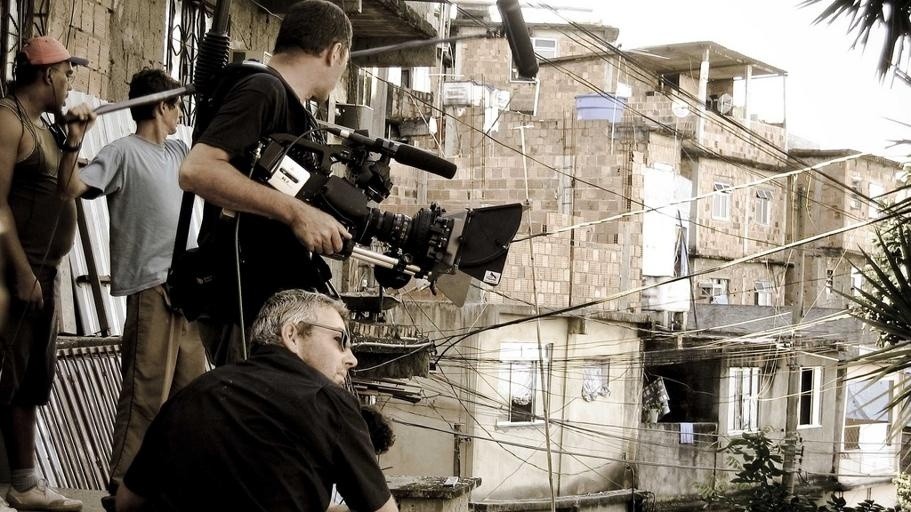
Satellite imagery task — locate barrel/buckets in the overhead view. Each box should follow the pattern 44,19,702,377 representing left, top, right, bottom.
649,409,659,423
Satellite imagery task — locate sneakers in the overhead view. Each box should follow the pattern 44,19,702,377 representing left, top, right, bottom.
6,483,83,511
0,496,17,512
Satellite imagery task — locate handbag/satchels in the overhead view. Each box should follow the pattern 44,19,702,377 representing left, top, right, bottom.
166,246,214,319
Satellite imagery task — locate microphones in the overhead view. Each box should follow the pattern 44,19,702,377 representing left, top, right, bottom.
496,0,539,78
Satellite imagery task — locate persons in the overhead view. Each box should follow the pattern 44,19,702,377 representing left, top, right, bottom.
113,288,402,511
326,403,397,511
177,0,356,368
1,35,86,510
56,67,212,512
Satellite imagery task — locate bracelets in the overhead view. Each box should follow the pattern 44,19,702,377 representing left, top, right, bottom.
62,141,82,153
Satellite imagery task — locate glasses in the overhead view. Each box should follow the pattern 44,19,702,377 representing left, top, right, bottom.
304,320,348,351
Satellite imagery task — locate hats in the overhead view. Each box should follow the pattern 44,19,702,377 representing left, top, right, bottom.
17,34,89,65
